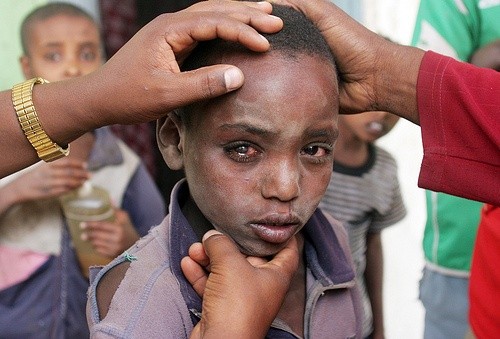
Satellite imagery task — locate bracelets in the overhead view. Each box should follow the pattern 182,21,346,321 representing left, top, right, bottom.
11,76,72,163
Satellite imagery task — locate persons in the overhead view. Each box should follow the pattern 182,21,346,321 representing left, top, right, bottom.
86,0,366,339
319,109,407,338
0,1,166,339
411,0,499,338
0,1,283,180
267,0,500,339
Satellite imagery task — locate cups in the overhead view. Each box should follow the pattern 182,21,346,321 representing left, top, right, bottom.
55,186,119,284
